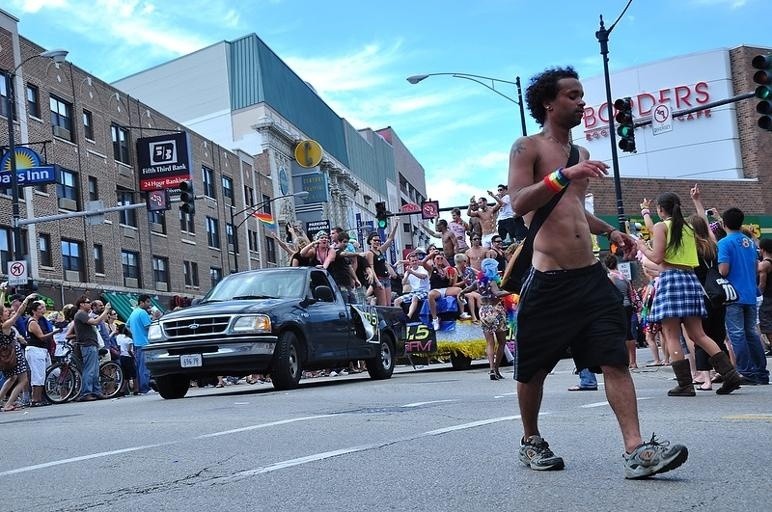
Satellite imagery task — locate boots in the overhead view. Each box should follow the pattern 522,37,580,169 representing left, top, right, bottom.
707,350,742,395
667,358,696,397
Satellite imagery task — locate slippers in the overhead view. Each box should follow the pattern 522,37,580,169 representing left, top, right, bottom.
692,378,705,385
246,379,257,384
567,384,598,391
215,383,226,388
696,384,712,390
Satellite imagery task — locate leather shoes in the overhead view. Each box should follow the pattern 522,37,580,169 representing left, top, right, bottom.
82,392,109,401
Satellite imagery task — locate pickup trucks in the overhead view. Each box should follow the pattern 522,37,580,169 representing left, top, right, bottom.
139,266,411,397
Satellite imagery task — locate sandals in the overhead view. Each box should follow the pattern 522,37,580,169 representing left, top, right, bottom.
489,368,506,380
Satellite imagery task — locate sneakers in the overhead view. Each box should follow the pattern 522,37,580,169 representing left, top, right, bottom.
459,312,472,320
518,435,565,471
622,440,688,480
764,350,772,359
754,374,770,386
432,315,440,331
739,375,758,386
328,370,349,376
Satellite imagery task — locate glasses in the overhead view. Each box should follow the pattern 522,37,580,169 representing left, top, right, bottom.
84,301,91,304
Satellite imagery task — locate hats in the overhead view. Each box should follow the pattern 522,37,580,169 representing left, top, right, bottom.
367,231,380,245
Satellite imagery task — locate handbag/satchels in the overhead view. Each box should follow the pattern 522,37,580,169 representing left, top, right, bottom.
624,276,643,313
498,235,534,296
0,340,18,372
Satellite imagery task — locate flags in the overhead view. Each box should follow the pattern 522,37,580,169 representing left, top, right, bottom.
251,210,275,226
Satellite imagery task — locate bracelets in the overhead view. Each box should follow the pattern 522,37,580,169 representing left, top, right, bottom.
544,166,569,194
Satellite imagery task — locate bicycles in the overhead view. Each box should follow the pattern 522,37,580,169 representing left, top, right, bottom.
42,338,126,406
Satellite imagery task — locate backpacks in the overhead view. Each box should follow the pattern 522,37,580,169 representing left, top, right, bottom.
699,256,740,309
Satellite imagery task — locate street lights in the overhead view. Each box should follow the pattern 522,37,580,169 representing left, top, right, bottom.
228,190,311,281
3,47,74,301
404,71,527,137
345,225,373,234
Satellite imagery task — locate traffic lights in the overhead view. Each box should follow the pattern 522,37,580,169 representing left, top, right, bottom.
375,201,388,229
179,179,193,216
614,96,635,156
610,242,622,254
752,50,772,133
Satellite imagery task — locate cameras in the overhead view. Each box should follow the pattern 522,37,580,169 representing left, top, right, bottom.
706,210,714,215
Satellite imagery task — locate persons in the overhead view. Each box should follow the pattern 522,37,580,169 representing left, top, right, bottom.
506,65,688,478
